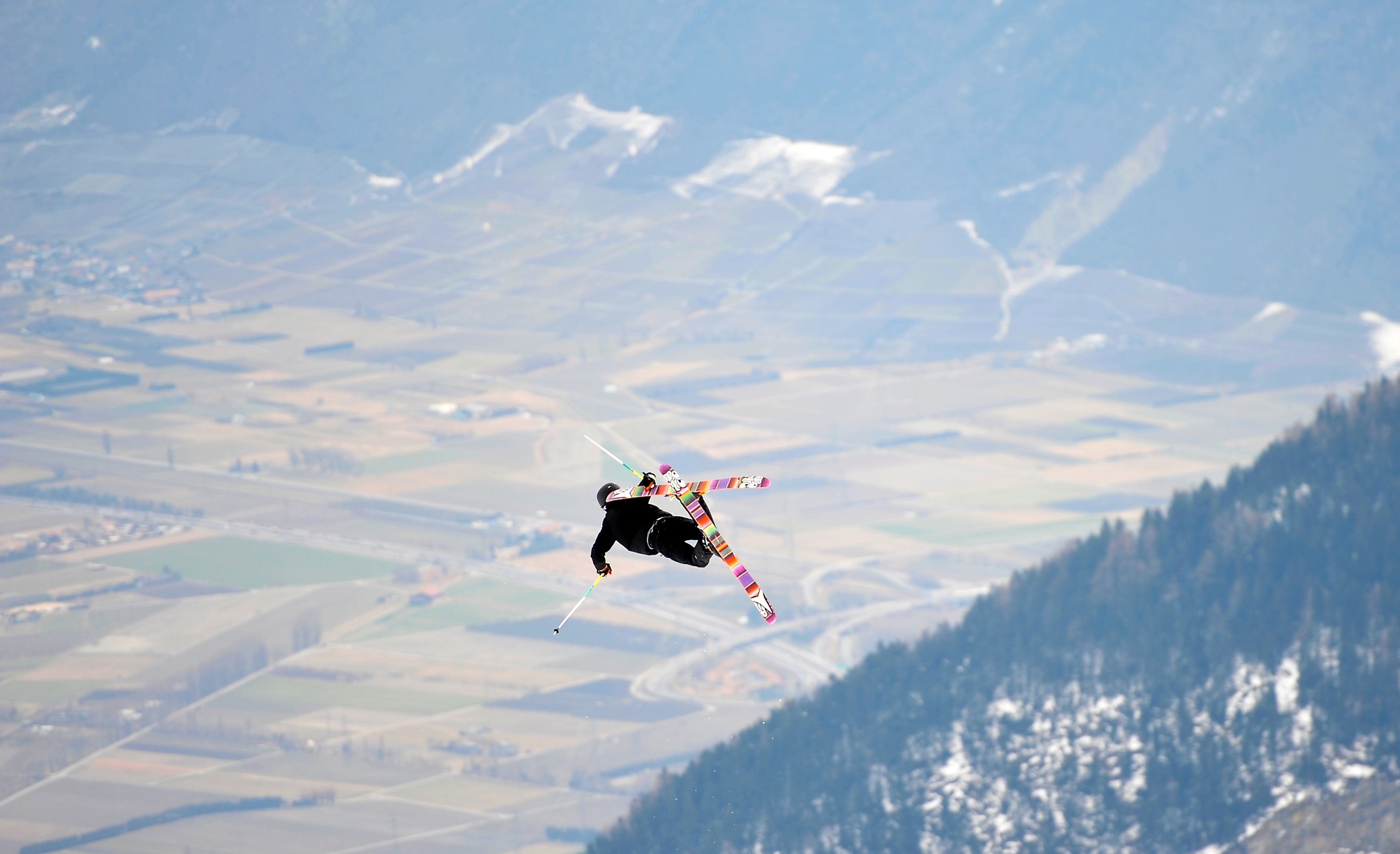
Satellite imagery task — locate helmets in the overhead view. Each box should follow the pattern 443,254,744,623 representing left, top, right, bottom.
597,483,619,507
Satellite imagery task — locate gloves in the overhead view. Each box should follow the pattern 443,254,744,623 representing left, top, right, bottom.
643,471,655,484
597,563,612,577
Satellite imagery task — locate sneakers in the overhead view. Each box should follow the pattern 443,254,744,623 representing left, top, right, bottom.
702,534,721,557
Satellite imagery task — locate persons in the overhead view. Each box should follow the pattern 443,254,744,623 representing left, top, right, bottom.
591,471,719,576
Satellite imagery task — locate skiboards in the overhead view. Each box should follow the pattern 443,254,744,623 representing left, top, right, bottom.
605,463,775,626
